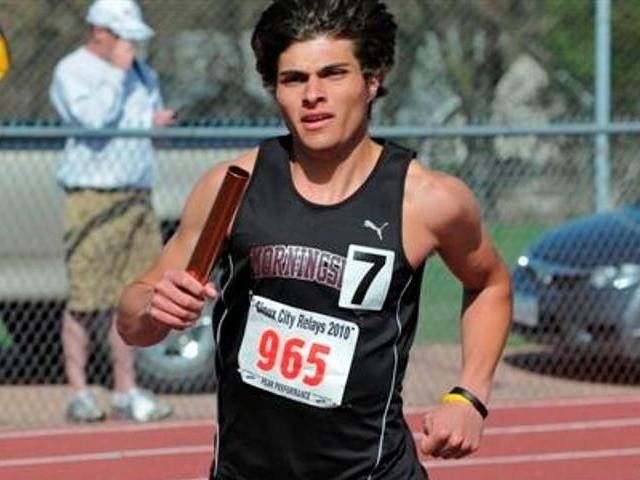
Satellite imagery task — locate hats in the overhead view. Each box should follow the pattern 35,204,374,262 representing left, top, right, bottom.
86,0,156,42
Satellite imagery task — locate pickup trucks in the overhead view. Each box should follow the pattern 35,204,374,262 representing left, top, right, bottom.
0,142,275,390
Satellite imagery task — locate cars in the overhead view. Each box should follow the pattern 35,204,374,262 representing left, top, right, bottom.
507,200,640,370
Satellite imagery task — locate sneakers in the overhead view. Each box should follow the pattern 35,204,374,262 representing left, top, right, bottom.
110,387,173,421
66,394,105,420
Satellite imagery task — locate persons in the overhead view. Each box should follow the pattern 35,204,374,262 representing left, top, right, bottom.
45,0,179,426
115,1,516,478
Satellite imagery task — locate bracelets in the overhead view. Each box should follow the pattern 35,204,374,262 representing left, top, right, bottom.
442,393,465,403
449,385,490,419
